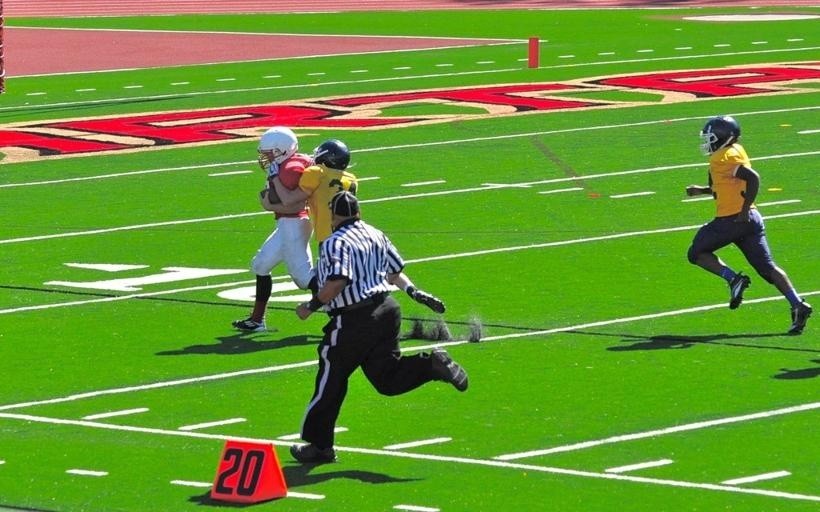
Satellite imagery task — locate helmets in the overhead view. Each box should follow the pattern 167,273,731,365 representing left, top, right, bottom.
313,139,350,170
699,116,741,156
257,126,298,169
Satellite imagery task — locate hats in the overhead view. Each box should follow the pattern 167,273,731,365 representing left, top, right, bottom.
332,191,359,217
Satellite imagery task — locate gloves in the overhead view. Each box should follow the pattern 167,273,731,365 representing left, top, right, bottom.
269,161,279,178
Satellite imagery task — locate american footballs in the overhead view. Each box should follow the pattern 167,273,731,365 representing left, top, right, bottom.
260,188,281,205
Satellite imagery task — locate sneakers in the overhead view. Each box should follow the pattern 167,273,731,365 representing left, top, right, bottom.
290,445,337,464
730,270,750,309
414,290,445,313
232,318,266,332
432,348,467,391
788,297,812,335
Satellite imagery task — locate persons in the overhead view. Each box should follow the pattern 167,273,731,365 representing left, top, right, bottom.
269,138,446,315
290,191,468,464
687,115,813,336
232,126,319,331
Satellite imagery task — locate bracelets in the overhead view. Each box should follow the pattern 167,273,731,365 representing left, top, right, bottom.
306,295,323,312
